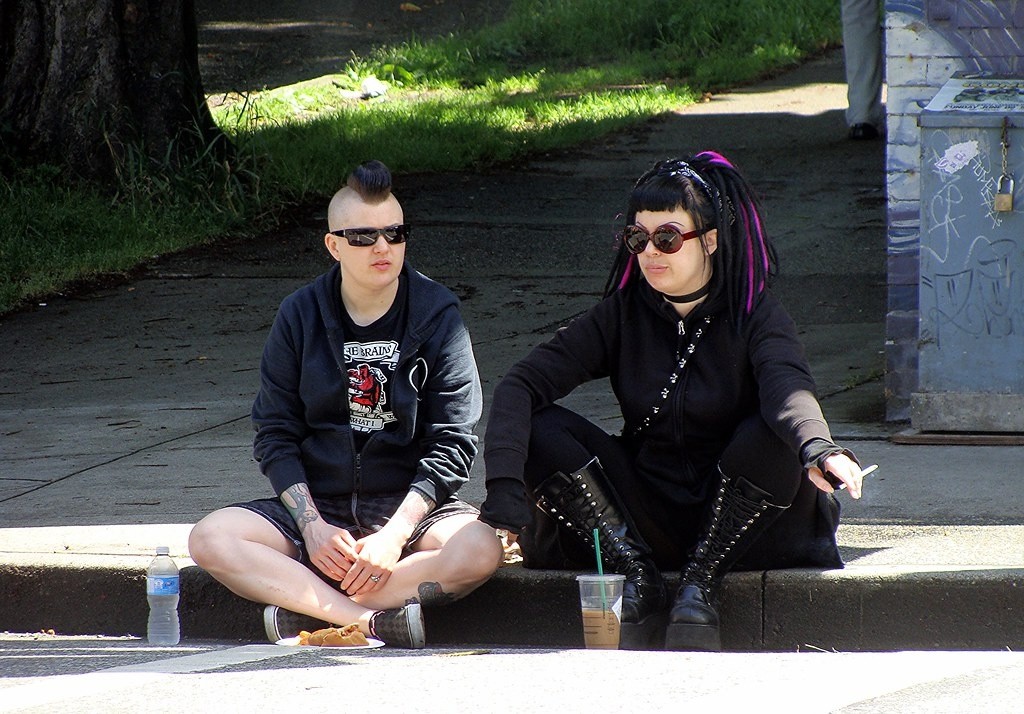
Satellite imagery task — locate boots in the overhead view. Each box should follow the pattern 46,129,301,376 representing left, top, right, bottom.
663,460,792,651
529,455,675,650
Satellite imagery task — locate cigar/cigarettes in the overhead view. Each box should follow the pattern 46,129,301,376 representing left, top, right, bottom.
839,463,880,490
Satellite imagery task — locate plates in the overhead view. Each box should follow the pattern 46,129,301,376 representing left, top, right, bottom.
275,636,384,649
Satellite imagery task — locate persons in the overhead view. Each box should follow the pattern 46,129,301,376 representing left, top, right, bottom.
186,162,507,652
475,153,864,653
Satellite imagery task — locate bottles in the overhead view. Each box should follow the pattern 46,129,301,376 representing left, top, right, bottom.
146,545,182,645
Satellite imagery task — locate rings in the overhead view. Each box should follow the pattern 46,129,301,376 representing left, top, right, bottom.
370,575,380,582
362,571,371,577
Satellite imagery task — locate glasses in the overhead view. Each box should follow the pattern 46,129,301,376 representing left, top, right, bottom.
330,223,411,247
623,224,714,255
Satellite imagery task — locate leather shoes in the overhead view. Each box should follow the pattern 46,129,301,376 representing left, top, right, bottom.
848,123,877,139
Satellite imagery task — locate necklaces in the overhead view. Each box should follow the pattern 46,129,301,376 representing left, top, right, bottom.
663,274,714,305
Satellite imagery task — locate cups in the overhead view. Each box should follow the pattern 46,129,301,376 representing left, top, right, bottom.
575,576,626,649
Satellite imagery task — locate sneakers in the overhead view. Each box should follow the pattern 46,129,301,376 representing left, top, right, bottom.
263,604,341,644
369,603,426,649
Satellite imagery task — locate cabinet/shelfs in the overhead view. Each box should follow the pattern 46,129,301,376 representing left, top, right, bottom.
909,69,1023,434
